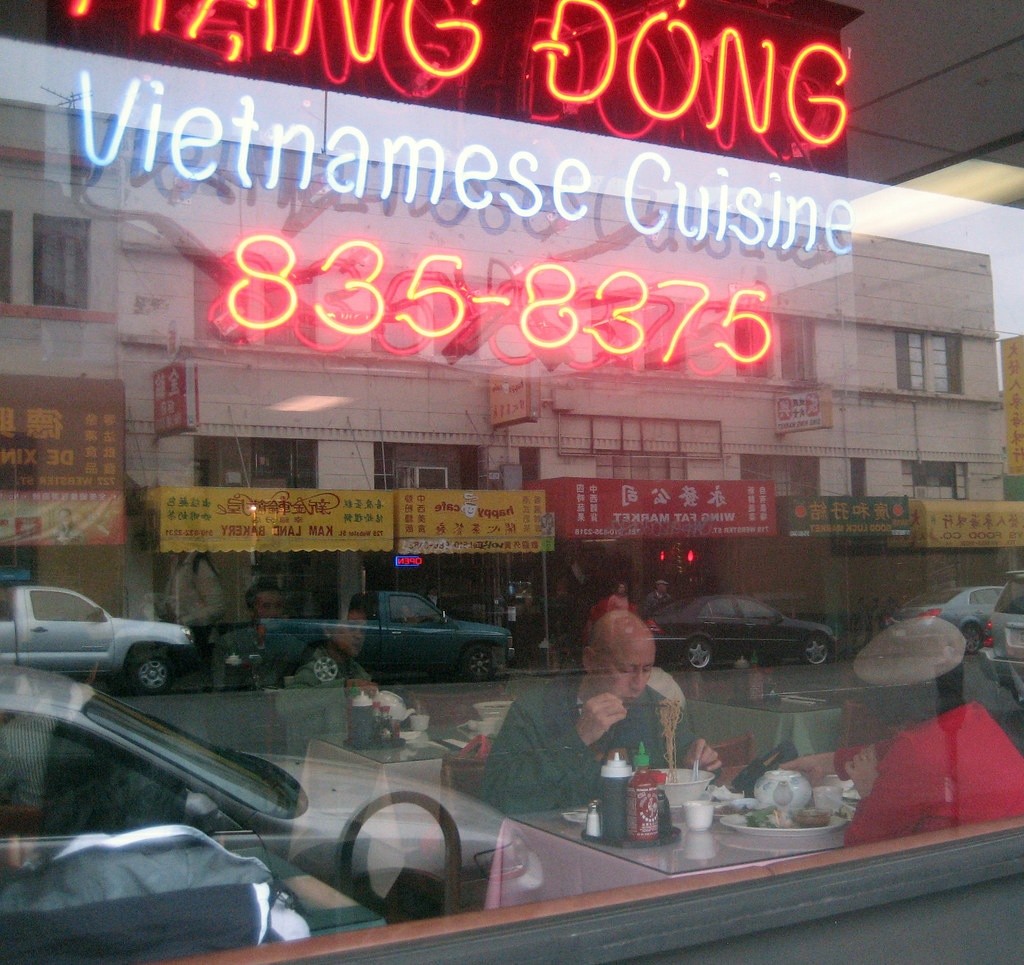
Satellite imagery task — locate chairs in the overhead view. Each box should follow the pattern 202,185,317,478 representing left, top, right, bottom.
441,761,517,918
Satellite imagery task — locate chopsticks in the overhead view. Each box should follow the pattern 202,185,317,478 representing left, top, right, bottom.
566,699,680,710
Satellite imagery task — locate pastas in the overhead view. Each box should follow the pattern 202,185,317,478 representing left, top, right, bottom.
652,698,683,783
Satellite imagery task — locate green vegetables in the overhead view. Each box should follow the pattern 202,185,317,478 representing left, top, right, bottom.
744,807,779,829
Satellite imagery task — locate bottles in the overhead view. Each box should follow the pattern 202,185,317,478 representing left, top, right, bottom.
585,801,602,836
599,751,633,839
351,691,400,744
625,741,659,842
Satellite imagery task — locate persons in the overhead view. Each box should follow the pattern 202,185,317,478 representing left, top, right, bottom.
775,616,1024,844
164,551,226,695
284,607,372,752
482,612,722,819
54,509,86,546
647,580,671,607
209,582,309,691
607,582,630,611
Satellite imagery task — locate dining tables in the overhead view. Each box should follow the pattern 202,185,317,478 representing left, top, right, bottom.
483,750,859,913
287,730,442,898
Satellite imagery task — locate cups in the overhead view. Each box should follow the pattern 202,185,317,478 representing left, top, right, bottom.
409,714,430,731
813,786,843,811
681,800,714,831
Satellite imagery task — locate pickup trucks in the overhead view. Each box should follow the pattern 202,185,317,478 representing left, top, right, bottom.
0,584,197,695
253,591,515,682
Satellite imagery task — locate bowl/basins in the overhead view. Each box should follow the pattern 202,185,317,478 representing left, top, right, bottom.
649,767,716,809
473,701,512,724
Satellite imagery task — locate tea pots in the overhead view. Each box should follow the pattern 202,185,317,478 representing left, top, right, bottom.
371,689,416,725
753,769,812,812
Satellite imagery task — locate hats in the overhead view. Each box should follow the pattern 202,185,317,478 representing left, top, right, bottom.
856,616,967,686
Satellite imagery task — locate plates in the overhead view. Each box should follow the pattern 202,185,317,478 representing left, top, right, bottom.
718,812,847,836
711,798,759,817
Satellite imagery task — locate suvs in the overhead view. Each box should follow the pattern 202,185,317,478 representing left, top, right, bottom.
977,570,1024,706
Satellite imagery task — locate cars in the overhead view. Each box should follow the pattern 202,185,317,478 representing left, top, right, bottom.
646,593,837,672
0,664,546,909
884,586,1003,656
